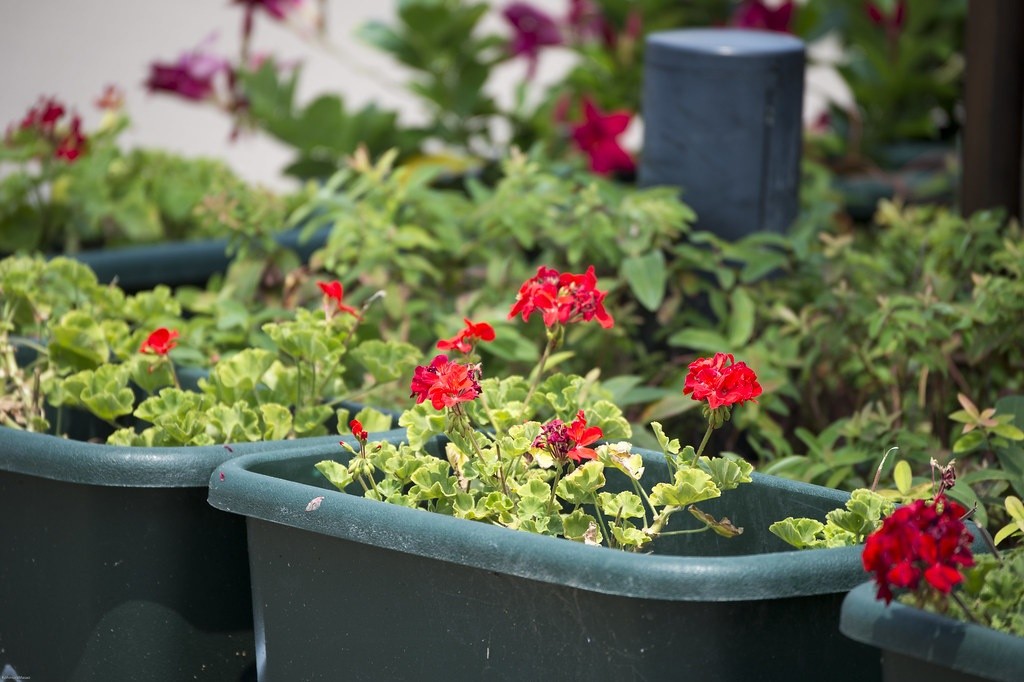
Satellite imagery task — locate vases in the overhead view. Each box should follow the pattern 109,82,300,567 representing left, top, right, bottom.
46,220,334,299
825,142,962,228
0,367,410,682
842,579,1024,681
206,419,979,682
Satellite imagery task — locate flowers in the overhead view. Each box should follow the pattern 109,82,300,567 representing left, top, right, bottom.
1,0,1024,638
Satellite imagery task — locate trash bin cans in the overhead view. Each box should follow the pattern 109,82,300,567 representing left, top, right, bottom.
641,27,807,242
